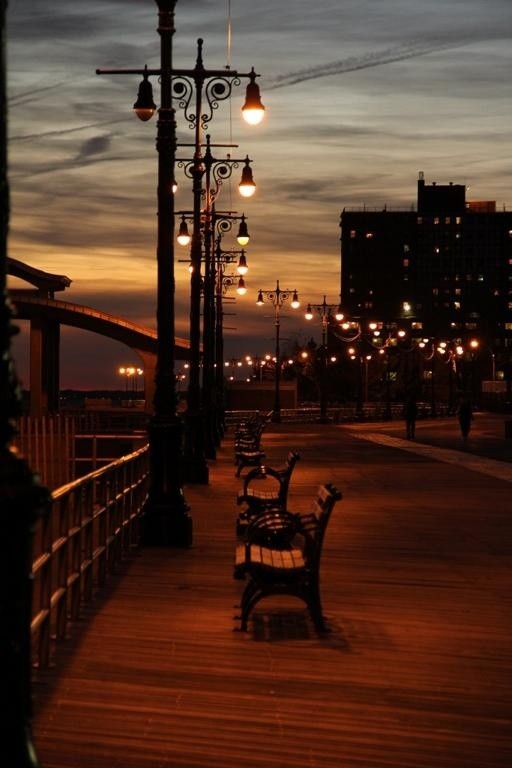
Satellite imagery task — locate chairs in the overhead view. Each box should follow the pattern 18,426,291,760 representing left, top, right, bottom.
232,407,275,476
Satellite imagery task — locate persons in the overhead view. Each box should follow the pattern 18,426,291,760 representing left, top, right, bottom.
455,380,476,442
404,379,418,440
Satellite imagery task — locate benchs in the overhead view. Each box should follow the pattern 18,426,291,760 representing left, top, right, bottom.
232,480,341,638
231,450,298,530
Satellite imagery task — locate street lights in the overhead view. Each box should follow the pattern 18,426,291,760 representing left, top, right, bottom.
199,264,247,430
96,39,266,478
173,191,250,449
190,236,249,441
173,133,256,462
